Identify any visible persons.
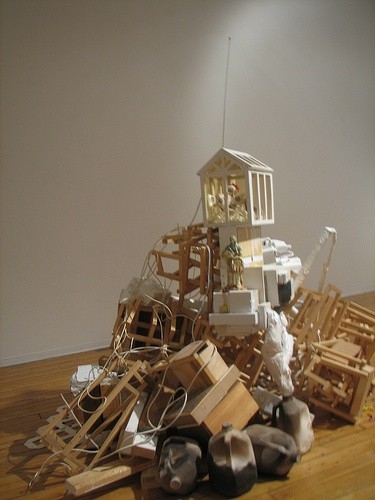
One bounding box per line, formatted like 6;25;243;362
222;235;245;291
